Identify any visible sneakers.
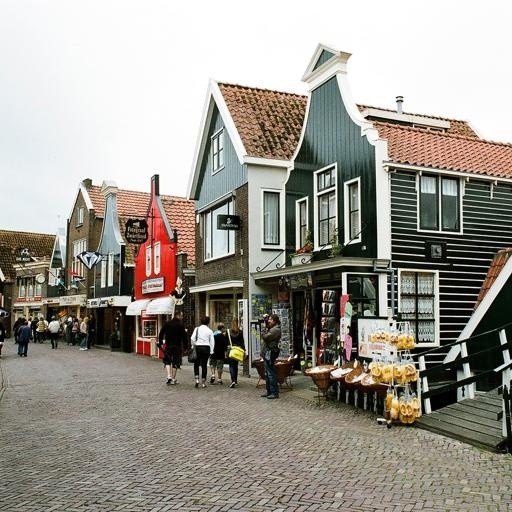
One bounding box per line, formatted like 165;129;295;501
195;377;238;388
165;377;177;384
79;347;88;351
260;394;279;399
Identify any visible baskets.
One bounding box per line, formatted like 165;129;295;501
305;362;376;393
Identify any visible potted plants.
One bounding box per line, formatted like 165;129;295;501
287;230;314;266
327;223;344;258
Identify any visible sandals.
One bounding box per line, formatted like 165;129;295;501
371;330;422;423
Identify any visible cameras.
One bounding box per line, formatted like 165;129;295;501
258;313;269;322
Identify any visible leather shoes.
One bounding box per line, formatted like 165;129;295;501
20;353;27;357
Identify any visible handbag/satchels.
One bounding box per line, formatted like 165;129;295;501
188;348;199;362
271;348;280;359
228;346;244;361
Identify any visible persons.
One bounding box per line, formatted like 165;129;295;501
159;310;189;385
261;314;281;399
0;312;96;359
169;277;186;319
191;317;245;388
